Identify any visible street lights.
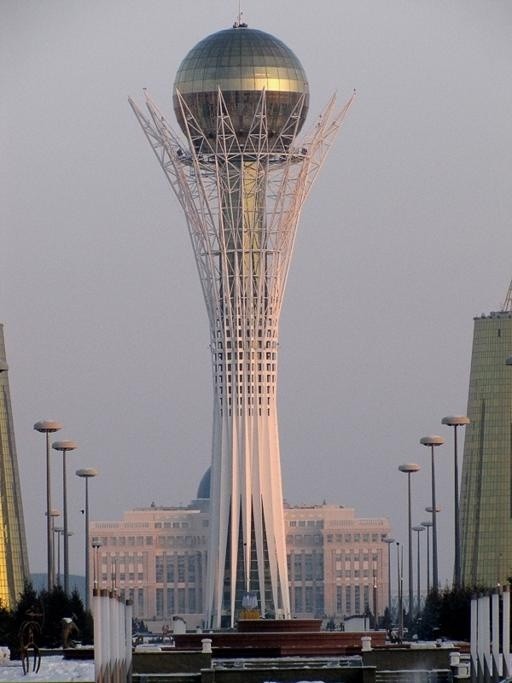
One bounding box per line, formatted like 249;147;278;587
33;420;98;612
381;415;469;633
